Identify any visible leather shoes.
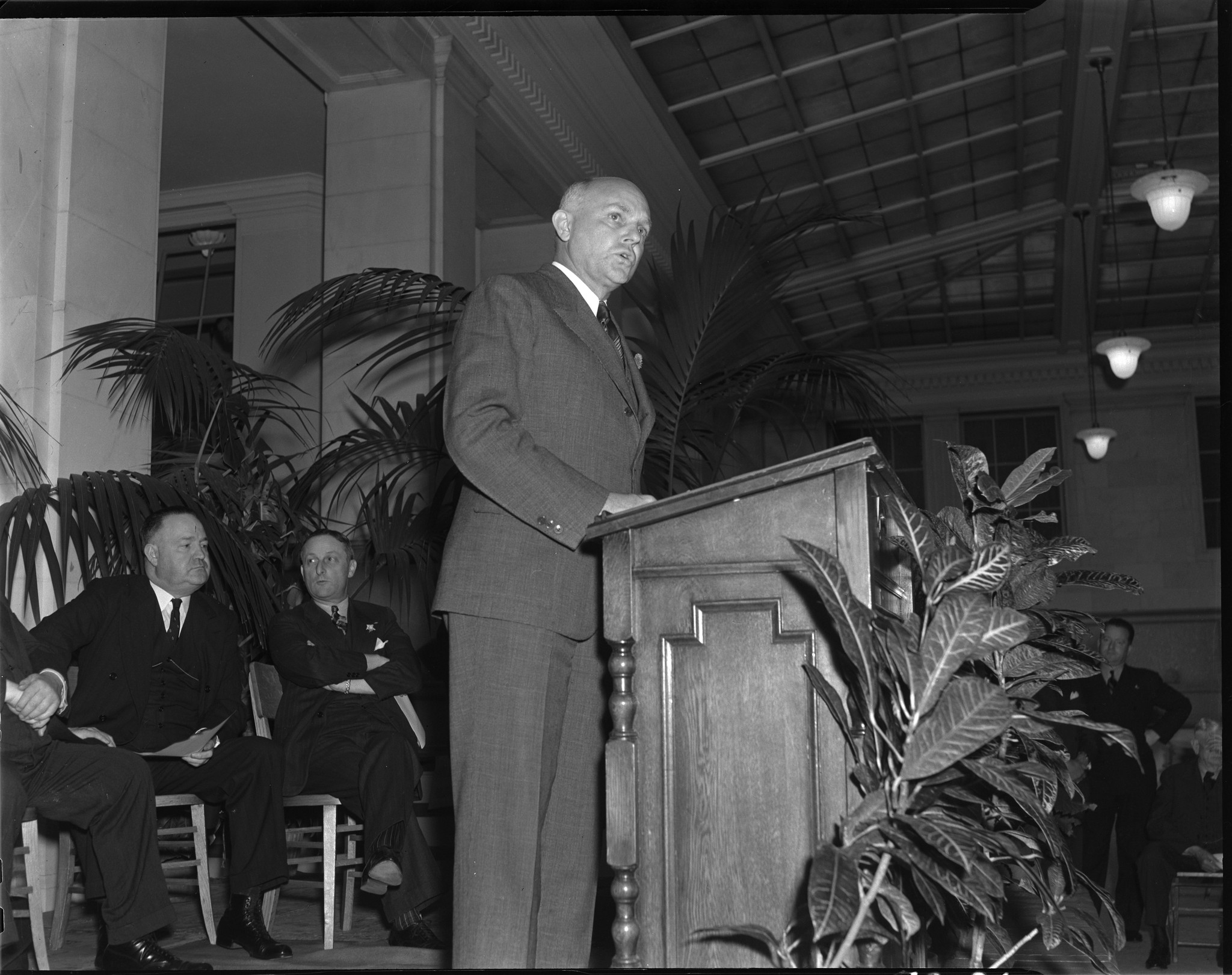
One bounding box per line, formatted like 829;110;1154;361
1145;943;1171;969
1125;930;1143;943
394;921;450;950
95;929;213;970
360;848;403;895
1216;946;1224;968
215;893;293;960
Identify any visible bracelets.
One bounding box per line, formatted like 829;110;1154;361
344;679;351;694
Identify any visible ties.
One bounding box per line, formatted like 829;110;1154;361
167;598;183;647
597;301;622;363
330;606;347;635
1204;771;1215;789
1106;670;1117;697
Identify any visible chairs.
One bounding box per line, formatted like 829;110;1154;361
246;657;366;953
9;804;50;970
45;664;218;950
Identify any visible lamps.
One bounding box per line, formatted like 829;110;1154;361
1127;0;1212;233
1088;54;1150;380
1067;202;1120;460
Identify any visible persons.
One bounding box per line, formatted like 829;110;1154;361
27;505;293;960
1141;718;1224;969
1062;618;1192;943
0;591;214;971
268;529;449;949
430;177;656;969
